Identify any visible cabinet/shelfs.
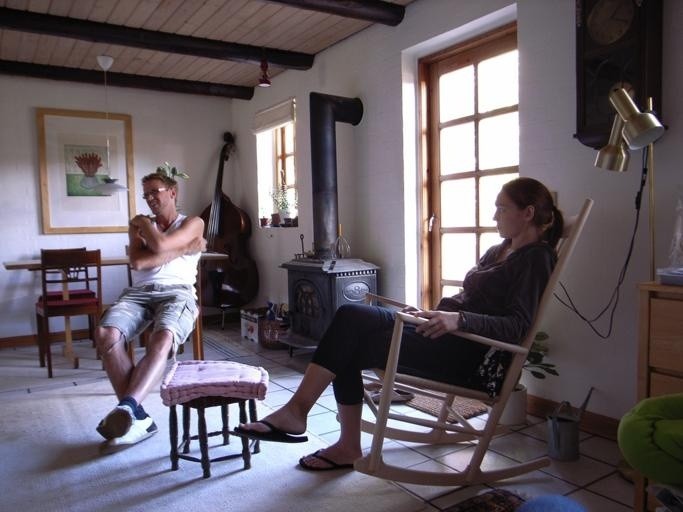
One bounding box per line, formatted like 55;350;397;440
634;278;682;512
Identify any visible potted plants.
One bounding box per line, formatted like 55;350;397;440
269;168;297;227
493;328;561;426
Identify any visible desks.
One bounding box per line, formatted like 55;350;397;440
2;249;230;378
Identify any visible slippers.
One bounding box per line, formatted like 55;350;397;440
234;420;308;443
299;449;353;471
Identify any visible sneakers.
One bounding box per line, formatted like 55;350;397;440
96;404;136;440
108;413;159;446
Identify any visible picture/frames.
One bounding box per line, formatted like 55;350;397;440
35;108;137;235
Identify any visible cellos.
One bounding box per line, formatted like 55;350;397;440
195;131;258;308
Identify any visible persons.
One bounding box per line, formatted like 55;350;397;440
92;172;208;448
234;174;565;471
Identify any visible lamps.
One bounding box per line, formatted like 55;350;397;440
258;59;271;87
594;85;664;483
87;56;130;196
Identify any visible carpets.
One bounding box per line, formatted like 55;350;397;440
440;489;525;512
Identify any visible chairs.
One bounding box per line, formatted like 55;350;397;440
32;246;102;378
40;247;92;342
335;191;592;490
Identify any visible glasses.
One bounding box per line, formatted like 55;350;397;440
143;187;169;199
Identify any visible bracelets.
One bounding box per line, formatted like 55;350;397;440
456;309;467;330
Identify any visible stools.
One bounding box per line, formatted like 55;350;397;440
168;395;260;478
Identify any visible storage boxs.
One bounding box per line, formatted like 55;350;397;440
239;303;288;349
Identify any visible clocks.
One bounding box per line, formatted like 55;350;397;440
572;2;665;152
